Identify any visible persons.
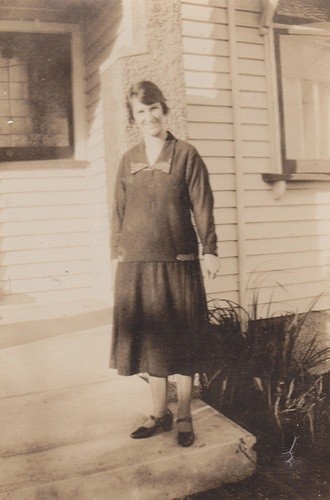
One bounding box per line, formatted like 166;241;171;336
109;81;222;448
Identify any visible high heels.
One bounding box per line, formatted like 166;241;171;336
130;409;173;439
176;418;194;447
